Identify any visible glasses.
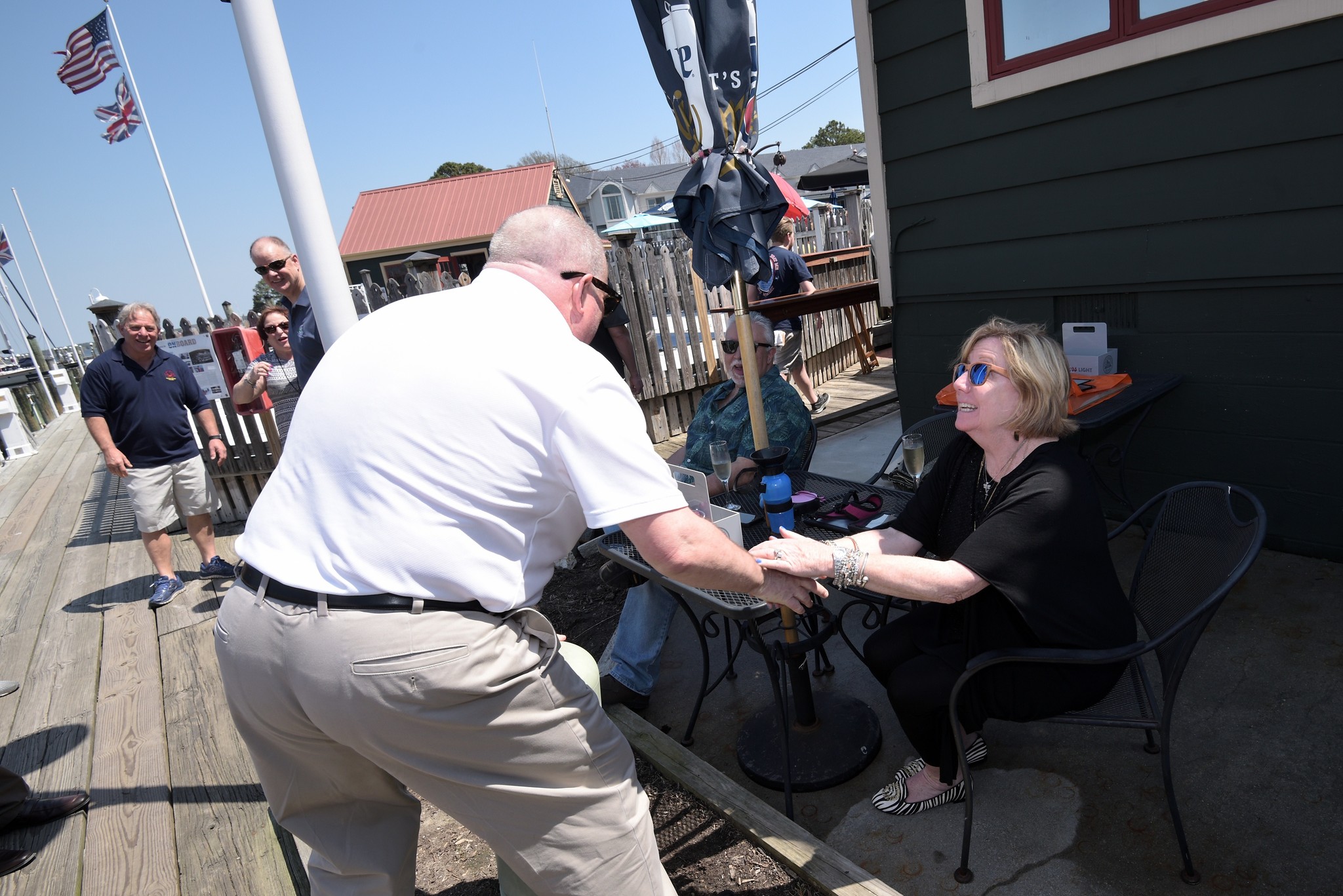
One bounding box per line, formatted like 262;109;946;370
263;321;290;334
953;362;1010;385
255;257;290;275
561;271;623;317
720;340;771;353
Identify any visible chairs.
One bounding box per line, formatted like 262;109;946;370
652;478;1268;888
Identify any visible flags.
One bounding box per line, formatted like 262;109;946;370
51;6;122;95
0;229;15;269
93;74;143;145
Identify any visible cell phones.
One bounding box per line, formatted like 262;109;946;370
732;509;764;527
847;510;900;534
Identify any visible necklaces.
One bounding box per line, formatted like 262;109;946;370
273;348;301;395
973;436;1031;534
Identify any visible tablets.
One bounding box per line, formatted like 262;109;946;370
803;516;856;536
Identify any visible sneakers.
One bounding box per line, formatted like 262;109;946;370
148;575;186;607
199;556;242;579
811;391;830;414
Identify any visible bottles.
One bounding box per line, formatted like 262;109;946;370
732;446;794;534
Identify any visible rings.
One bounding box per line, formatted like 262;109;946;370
774;547;781;560
257;368;259;372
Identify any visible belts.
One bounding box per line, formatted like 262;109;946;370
239;562;488;613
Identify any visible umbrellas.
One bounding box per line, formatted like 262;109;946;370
630;0;813;484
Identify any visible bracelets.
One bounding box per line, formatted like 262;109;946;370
821;536;870;590
245;372;256;386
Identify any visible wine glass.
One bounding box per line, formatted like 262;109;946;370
902;433;925;492
710;440;741;511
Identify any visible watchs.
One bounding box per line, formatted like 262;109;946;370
208;434;223;441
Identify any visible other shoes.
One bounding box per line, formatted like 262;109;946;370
599;559;649;588
871;772;974;815
895;736;988;781
599;674;648;712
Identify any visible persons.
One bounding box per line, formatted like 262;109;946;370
745;217;828;414
79;300;244;608
583;295;644;394
249;234;325;394
747;315;1137;818
231;304;302;463
598;310;817;710
211;205;829;895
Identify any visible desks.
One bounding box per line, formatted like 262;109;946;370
596;470;916;796
710;278;880;374
929;367;1187;545
